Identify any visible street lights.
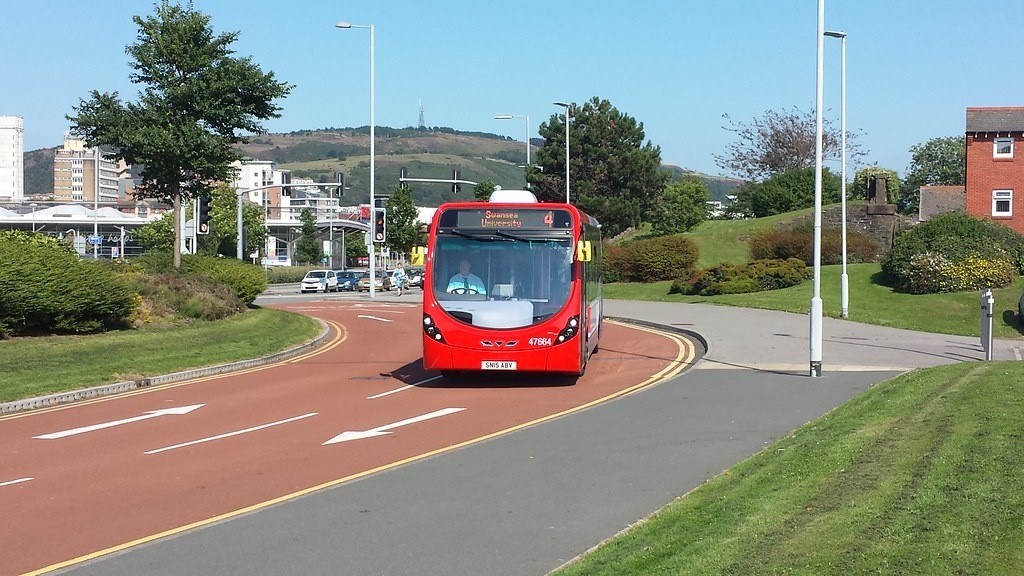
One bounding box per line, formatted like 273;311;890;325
493;115;531;193
825;30;849;320
332;228;344;269
29;203;37;232
336;22;377;299
553;102;571;203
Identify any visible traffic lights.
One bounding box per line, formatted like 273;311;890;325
196;195;212;235
373;208;386;243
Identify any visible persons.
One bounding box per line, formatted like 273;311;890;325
446;259;486;294
392;263;405;296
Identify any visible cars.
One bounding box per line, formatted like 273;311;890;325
357;270;392;293
335;271;365;291
387;267;424;290
300;270;338;294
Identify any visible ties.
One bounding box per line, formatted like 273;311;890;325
462;277;470;294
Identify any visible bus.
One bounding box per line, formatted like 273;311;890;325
412;190;604;379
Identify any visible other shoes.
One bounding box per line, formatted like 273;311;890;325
398;293;401;296
